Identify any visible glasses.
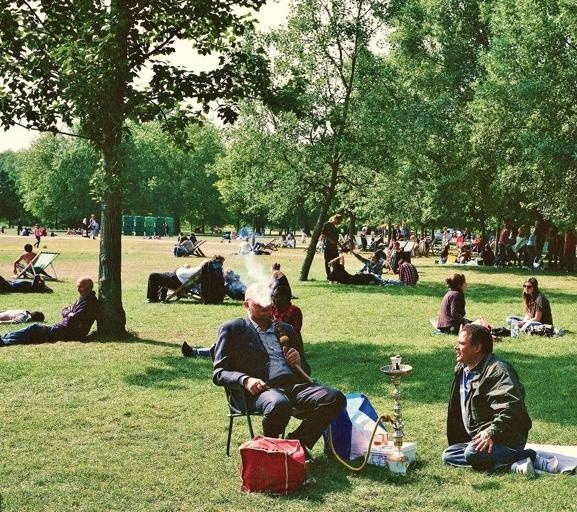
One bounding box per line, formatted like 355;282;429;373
523;285;533;288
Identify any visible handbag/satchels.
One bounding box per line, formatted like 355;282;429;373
239;434;305;496
323;393;389;471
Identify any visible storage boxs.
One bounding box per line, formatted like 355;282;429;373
364;441;416;466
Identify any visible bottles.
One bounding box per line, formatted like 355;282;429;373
511;320;519;337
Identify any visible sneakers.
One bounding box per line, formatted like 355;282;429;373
511;457;535;476
182;342;192;357
534;453;559;474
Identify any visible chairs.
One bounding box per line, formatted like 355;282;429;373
264;239;278;250
159;260;223;303
16;252;59;280
382;237;480;270
211;344;329;458
185;240;205;256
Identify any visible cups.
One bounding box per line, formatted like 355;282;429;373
380;433;389;447
390;357;403;370
373;435;383;446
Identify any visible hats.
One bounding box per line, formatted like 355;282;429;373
245;282;270;301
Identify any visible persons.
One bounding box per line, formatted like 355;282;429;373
442;227;451;251
67;228;76;235
360;227;382;251
147;254;225;302
83;215;99;239
162;223;169;236
327;255;384;285
268;262;289;286
456;230;496;266
0;307;44;324
302;230;307;244
318;214;348;276
223;225;296;254
17;221;30;236
353;242;418;285
495;224;542;270
14;244;48;276
441;322;559;475
436;272;514;336
33;224;47;248
391;222;430;257
504;277;554;334
211;285;345;460
549;229;574;270
173;233;197;257
182;284;302;356
0;276;96;347
